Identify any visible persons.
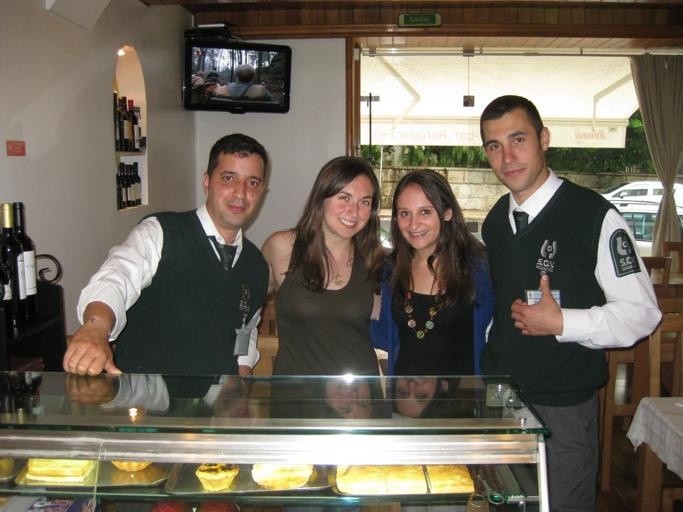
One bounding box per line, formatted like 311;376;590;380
63;133;270;376
214;65;272;100
260;156;386;377
192;71;204;102
64;377;269;434
392;376;462;418
369;168;496;374
482;95;663;512
309;378;386;418
196;66;220;101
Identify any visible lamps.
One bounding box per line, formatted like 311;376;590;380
462;51;475;108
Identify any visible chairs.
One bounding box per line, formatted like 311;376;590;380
639;317;683;512
599;296;683;441
663;242;681;275
638;256;671;297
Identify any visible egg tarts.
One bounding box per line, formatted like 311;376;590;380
111;466;164;484
197;460;239;492
114;460;151;472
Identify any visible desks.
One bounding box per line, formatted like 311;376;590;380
598;312;683;492
624;396;683;511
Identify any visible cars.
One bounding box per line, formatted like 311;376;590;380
562;200;683;258
600;182;683;215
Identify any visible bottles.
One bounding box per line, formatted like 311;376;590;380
0;260;12;328
116;163;141;210
111;93;141;153
0;371;42;424
0;204;28;329
8;204;37;323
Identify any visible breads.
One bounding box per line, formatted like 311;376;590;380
335;462;427;498
27;459;99;483
424;463;474;494
251;462;313;491
0;458;16;477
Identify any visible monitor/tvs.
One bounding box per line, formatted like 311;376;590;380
184;39;292;115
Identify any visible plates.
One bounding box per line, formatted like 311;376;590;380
329;464;481;500
14;464;170;488
164;463;337;497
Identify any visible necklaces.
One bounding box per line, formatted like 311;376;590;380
393;245;452;339
324;262;352;287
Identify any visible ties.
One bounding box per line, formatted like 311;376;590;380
207;235;238;272
512;210;529;234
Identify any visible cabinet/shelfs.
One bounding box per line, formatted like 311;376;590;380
1;372;549;512
